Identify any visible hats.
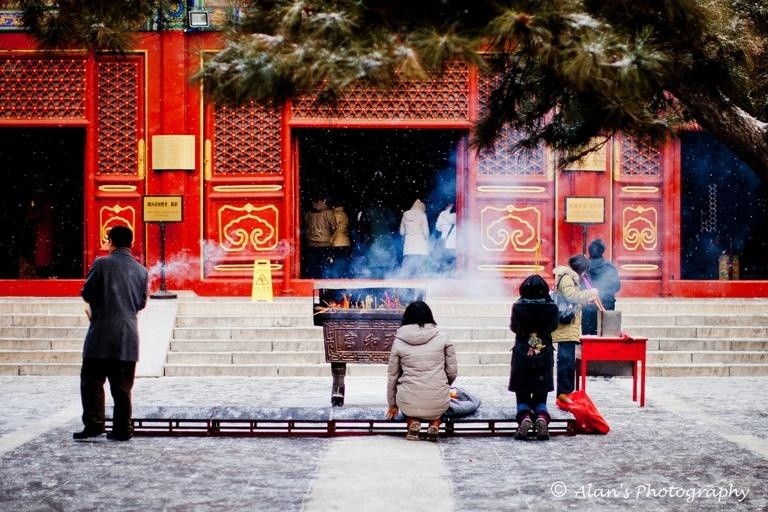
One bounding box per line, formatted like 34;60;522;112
413;200;426;212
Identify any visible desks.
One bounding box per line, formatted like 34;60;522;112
572;333;651;411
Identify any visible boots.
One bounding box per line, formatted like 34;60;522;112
534;410;550;440
426;420;441;442
514;409;534;439
407;417;421;440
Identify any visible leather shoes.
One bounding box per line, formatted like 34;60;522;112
73;428;132;441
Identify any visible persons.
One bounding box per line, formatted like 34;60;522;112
509;275;558;439
550;254;596;397
386;301;457;441
73;226;149;441
581;239;621;380
1;202;84;280
301;193;456;279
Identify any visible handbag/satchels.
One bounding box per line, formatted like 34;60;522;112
430;238;445;261
450;386;481;414
556;390;610;434
550;287;577;325
